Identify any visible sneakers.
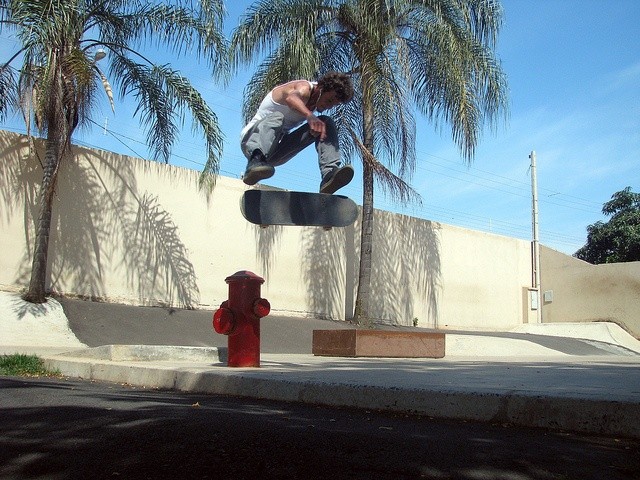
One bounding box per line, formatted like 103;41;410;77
243;156;274;184
320;165;354;194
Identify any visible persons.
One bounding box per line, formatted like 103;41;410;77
239;72;354;194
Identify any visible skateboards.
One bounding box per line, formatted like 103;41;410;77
239;189;358;231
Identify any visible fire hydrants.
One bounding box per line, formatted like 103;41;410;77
213;271;270;367
